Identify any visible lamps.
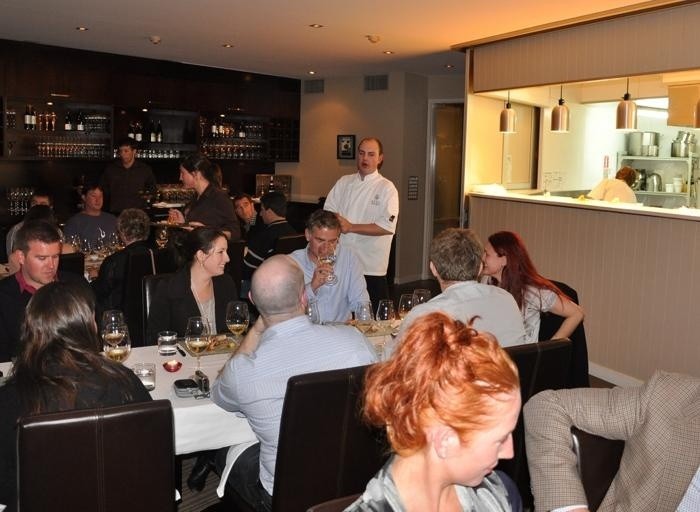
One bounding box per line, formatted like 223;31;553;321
551;84;570;132
615;76;637;129
499;89;519;134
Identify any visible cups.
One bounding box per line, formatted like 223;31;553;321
666;178;683;193
157;329;178;358
399;289;430;322
134;364;157;392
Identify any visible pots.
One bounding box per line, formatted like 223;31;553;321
628;132;659;157
671;143;696;157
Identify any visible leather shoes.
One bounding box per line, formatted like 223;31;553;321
187;455;215;491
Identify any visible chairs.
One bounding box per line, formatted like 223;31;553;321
112;233;306;347
571;425;625;512
14;400;176;512
221;364;391;512
57;252;84;280
502;280;590;458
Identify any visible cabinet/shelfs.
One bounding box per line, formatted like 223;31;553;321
617;154;692;207
0;96;114;163
201;112;270;160
115;105;201;158
271;117;300;161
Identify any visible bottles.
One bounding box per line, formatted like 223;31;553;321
269;176;275;192
282;184;289;201
7;103;264;161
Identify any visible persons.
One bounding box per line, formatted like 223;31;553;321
168;152;241;241
203;255;383;511
588;167;639;202
323;137;399;300
483;231;584;340
7;186;74;258
395;228;522;356
0;281;155;511
90;208;169;346
64;183;118;249
148;225;238;344
523;371;700;512
345;311;523;512
240;192;299;279
0;220;96;362
99;137;158;216
293;210;374;326
234;195;265;236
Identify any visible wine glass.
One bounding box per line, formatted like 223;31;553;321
305;303;320;324
376;299;396;346
184;317;211;380
102;310;130;365
34;141;264;161
317;242;338;286
8;190;34;215
354;302;373;347
225;302;250;361
71;229;122;259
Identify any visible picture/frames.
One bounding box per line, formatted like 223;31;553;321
337;135;355;159
